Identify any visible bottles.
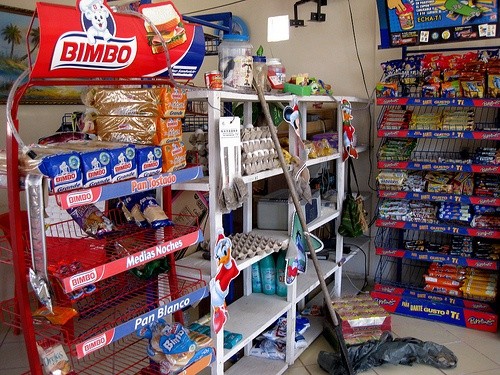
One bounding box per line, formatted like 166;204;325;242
266;57;287;95
251;55;268;95
259;254;276;295
217;34;254;94
251;262;263;293
276;248;287;297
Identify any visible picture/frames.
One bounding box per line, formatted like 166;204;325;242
0;4;90;106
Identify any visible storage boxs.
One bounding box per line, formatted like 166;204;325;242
323;294;392;355
256;188;322;232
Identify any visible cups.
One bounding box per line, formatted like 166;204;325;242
204;70;224;91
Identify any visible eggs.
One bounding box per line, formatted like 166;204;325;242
187;125;287;261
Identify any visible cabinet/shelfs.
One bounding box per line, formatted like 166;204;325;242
169;94;373;374
371;47;500;334
0;79;217;375
